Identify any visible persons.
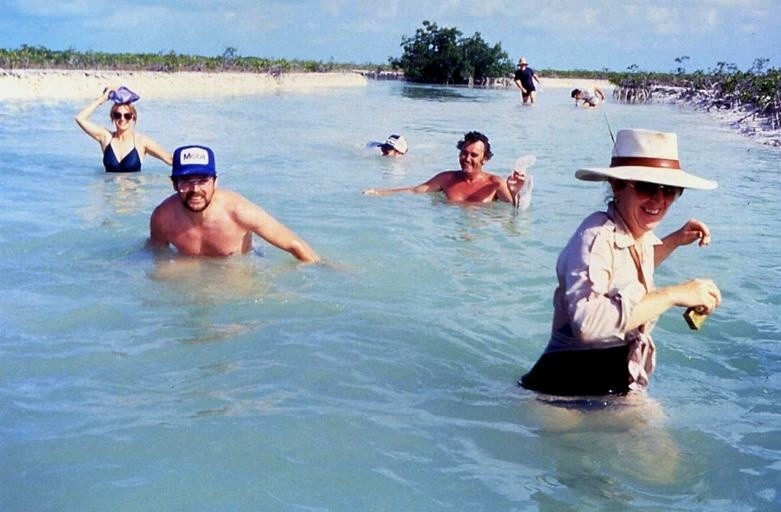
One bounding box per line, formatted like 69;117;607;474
516;126;720;487
363;133;531;215
143;146;325;264
375;136;410;157
75;90;178;172
571;86;609;108
516;58;542;105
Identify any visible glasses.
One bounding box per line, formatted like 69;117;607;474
113;111;133;120
624;181;685;202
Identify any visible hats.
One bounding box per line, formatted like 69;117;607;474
171;146;216;178
517;58;528;66
377;134;406;155
575;128;719;191
